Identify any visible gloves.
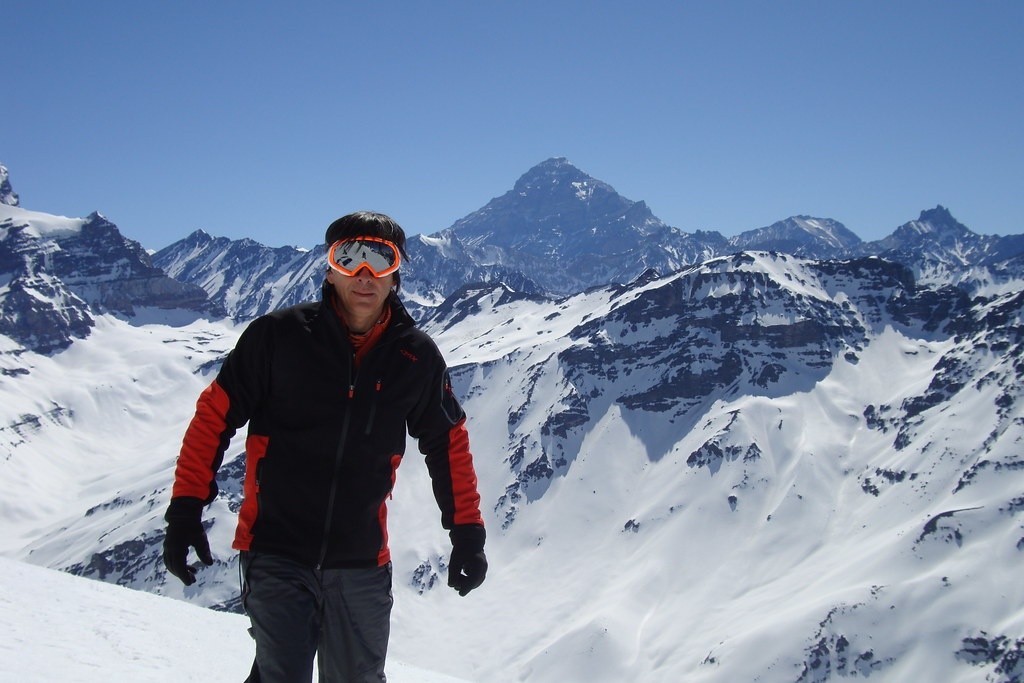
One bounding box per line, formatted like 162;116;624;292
162;502;213;586
448;525;488;596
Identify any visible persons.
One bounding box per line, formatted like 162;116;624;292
163;211;487;683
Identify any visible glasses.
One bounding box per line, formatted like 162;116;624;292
328;235;401;278
